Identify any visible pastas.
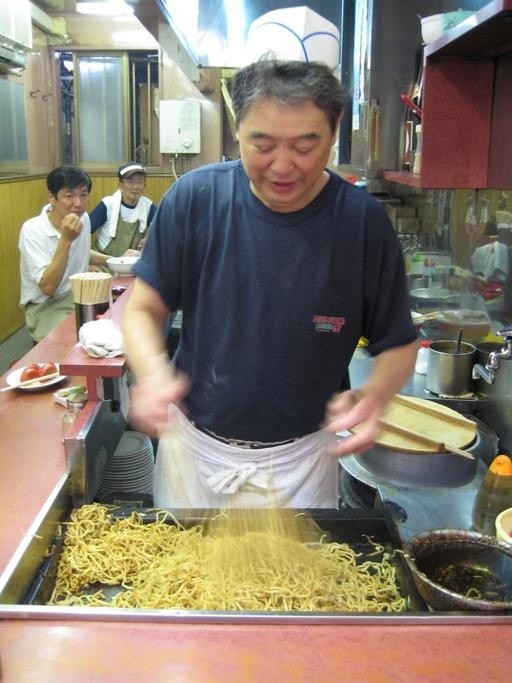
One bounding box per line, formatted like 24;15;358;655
46;502;408;613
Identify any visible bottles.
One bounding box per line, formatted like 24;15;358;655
416;338;429;376
410;256;424;273
471;453;512;534
402;46;420;171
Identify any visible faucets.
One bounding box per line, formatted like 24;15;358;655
472;330;512;383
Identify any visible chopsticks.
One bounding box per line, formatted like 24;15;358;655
0;372;62;392
69;272;113;305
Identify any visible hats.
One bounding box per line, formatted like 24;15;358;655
246;7;342;71
118;163;146;179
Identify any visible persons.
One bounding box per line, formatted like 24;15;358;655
88;161;158;274
122;57;422;507
19;167;101;345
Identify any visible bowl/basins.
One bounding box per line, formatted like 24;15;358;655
404;527;512;610
420;9;470;45
106;256;141;276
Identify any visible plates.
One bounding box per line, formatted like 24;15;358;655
6;364;70;394
96;430;154;495
